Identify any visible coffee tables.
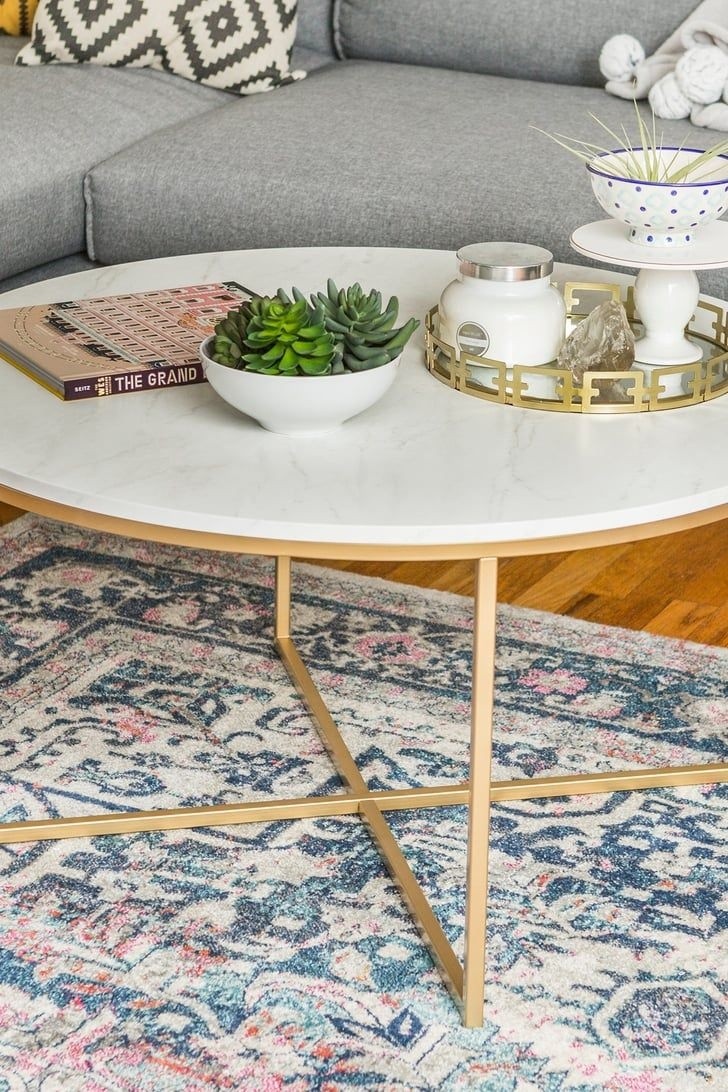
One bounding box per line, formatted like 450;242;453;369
0;247;728;1027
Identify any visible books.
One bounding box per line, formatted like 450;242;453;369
0;281;264;401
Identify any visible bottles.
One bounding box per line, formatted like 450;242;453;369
438;242;564;370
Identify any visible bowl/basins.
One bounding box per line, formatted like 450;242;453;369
585;145;728;245
200;335;405;436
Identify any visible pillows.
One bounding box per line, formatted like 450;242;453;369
0;0;307;94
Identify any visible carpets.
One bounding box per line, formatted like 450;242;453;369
0;510;728;1092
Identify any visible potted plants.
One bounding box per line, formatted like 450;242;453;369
199;277;420;433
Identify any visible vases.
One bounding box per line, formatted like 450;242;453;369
585;148;728;247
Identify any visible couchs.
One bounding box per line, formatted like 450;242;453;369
0;0;728;302
